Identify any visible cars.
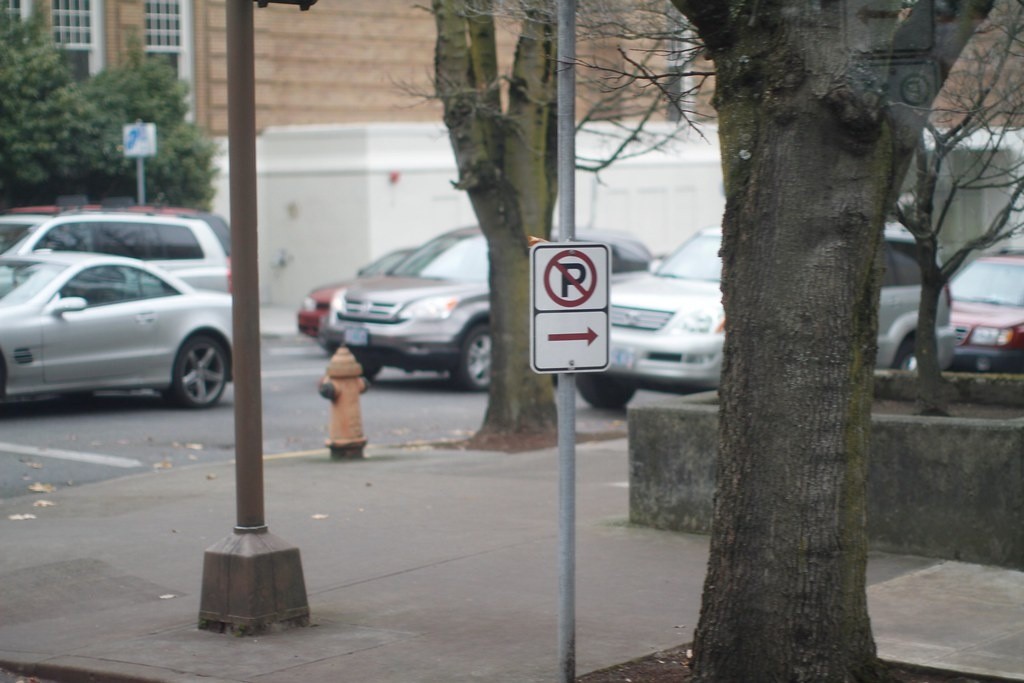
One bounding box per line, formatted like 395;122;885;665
948;256;1023;372
1;251;232;408
316;227;650;392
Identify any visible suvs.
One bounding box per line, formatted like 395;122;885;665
1;213;232;293
573;224;955;407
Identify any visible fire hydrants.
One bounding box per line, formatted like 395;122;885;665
319;342;371;456
